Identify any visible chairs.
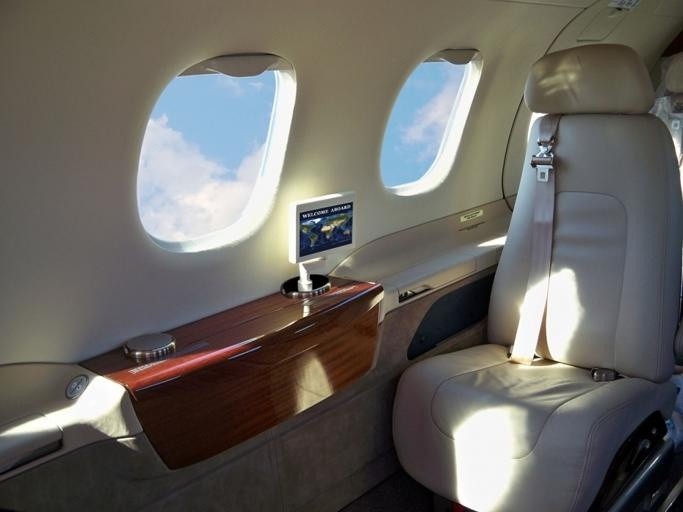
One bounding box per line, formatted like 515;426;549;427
648;52;683;198
393;43;683;512
